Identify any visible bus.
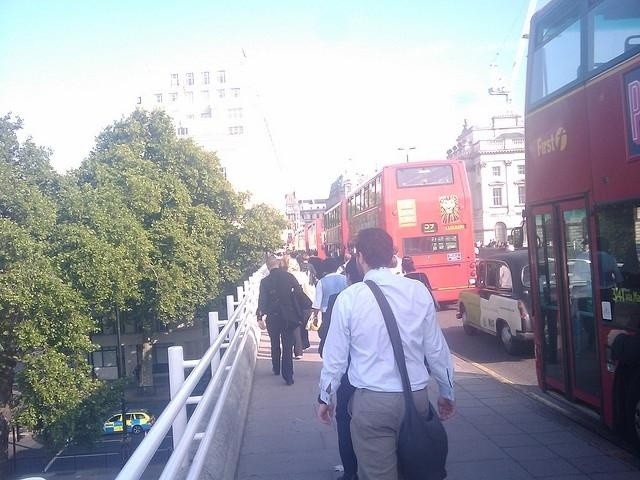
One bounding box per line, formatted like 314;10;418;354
288;160;478;306
525;0;640;449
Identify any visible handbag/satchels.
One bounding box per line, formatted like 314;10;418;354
395;400;448;480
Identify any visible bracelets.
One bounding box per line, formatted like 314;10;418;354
314;316;317;320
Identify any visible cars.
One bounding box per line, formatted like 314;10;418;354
103;408;156;435
455;250;536;355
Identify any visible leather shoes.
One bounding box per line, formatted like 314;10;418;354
337;473;358;480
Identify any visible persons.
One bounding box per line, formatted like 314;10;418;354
605;328;639;362
474;239;508;255
287;257;310;360
278;246;353;288
574;235;624;342
313;259;348;328
620;247;640;289
255;254;303;385
313;223;457;480
402;256;441;312
318;257;365;480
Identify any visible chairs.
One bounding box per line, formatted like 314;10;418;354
577;33;640;79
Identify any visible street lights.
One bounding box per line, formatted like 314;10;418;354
398;146;416;162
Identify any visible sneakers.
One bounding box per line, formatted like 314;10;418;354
272;352;304;386
